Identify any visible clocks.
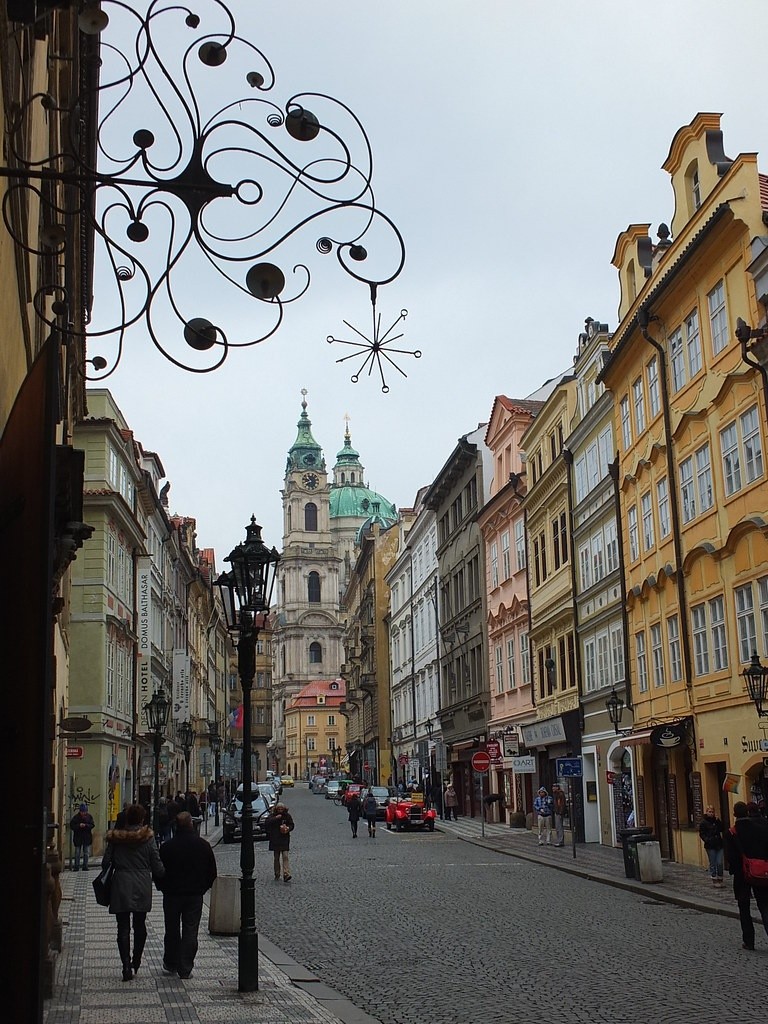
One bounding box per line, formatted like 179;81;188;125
302;472;320;489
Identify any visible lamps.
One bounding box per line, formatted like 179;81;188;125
738;651;768;718
604;684;626;735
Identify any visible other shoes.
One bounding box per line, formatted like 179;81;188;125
72;868;79;872
554;843;563;846
82;868;90;871
163;963;178;973
179;974;193;979
741;942;754;950
284;875;292;882
275;874;280;880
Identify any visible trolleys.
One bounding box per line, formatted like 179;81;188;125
334;789;346;806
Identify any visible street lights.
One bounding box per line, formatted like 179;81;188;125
209;511;282;994
336;744;342;771
330;746;337;762
211;734;225;826
176;717;198;794
140;683;172;881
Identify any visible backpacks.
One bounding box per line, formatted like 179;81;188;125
367;797;376;811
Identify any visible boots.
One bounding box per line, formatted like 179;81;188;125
711;876;721;887
717;878;726;887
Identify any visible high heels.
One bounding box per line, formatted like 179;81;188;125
130;958;141;974
122;966;132;982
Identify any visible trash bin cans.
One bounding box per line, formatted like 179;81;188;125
620;827;664;884
207;873;241;936
192;817;202;837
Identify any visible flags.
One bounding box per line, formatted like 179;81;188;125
228;705;243;728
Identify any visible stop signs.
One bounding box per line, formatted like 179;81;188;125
471;751;492;773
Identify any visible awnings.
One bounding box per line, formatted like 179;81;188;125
619;722;682;748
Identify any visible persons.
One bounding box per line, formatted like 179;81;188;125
482;792;506;823
70;804;95;872
532;786;555;846
726;801;768;951
698;804;726;887
265;802;295;882
552;783;567;846
388;774;459;821
152;812;218;978
347;794;362;838
363;794;378;838
145;774;238;845
101;804;166;982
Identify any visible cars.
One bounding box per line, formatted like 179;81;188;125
308;773;345;799
344;784;399;819
220;781;279;843
266;768;295;795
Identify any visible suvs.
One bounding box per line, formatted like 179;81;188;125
384;790;437;832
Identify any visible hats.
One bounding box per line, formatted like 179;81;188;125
125;804;146;818
79;804;89;813
351;794;358;798
746;802;758;811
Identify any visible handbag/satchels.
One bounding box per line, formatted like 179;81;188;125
92;860;114;907
743;858;768;884
